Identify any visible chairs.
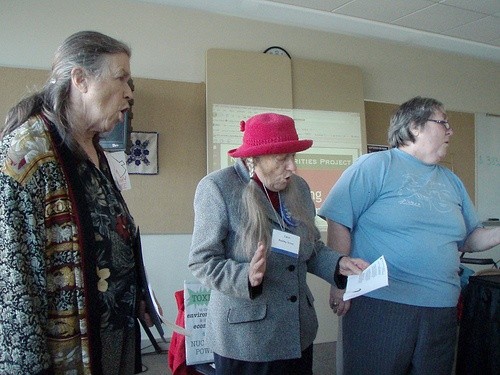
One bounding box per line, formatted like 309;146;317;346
174;289;216;375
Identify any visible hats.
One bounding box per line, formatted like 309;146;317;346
228;113;313;157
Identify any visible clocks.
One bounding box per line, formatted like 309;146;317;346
263;46;291;59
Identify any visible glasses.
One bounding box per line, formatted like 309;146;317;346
429;119;449;130
